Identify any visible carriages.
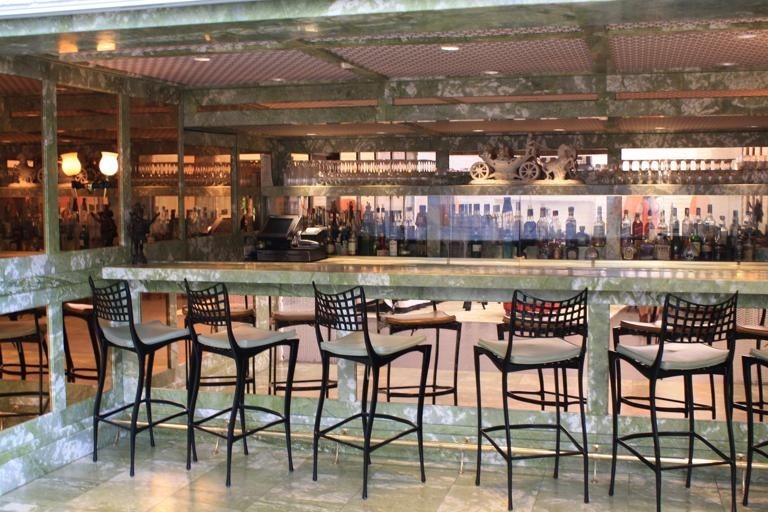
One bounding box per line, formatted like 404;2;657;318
13;152;91;185
471;136;577;180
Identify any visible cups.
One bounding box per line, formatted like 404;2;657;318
638;305;659;323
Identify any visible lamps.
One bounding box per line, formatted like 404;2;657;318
59;150;82;177
99;150;119;177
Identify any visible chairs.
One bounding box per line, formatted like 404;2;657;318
88;275;203;476
312;281;433;501
472;287;590;509
609;290;765;512
0;301;101;429
184;294;588;414
182;278;299;487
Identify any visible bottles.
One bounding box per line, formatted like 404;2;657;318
299;200;426;257
442;197;606;263
621;197;768;262
1;196;263;256
742;147;768;169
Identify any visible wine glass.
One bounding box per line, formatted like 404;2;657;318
128;160;261;187
579;158;737;185
279;156;469;187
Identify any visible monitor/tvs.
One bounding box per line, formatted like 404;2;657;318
258;214;302;241
